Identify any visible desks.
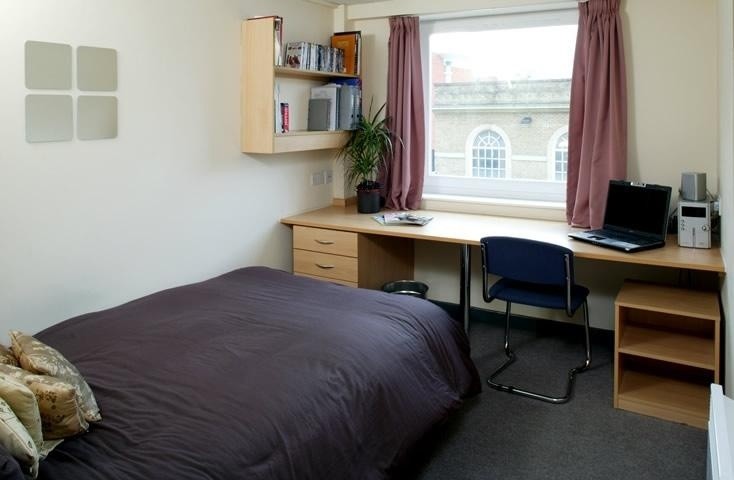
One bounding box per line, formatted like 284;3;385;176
280;203;726;288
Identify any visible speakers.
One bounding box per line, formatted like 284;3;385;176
683;174;707;202
678;202;711;248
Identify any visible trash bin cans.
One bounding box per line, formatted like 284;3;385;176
382;278;431;297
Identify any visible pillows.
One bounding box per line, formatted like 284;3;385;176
0;395;40;479
9;329;103;423
0;343;19;367
0;376;44;447
1;362;90;441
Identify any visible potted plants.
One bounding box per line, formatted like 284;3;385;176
334;93;408;215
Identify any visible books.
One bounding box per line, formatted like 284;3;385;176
373;212;433;227
245;16;361;76
273;79;362;133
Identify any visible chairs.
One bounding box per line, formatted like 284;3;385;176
478;235;595;403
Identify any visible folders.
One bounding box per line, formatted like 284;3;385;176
247;14;284;67
340;85;360;131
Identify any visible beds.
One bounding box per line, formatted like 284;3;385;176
1;265;483;479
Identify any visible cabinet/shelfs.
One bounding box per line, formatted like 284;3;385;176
241;17;364;155
612;279;722;430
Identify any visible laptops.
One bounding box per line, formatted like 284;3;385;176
569;180;671;254
